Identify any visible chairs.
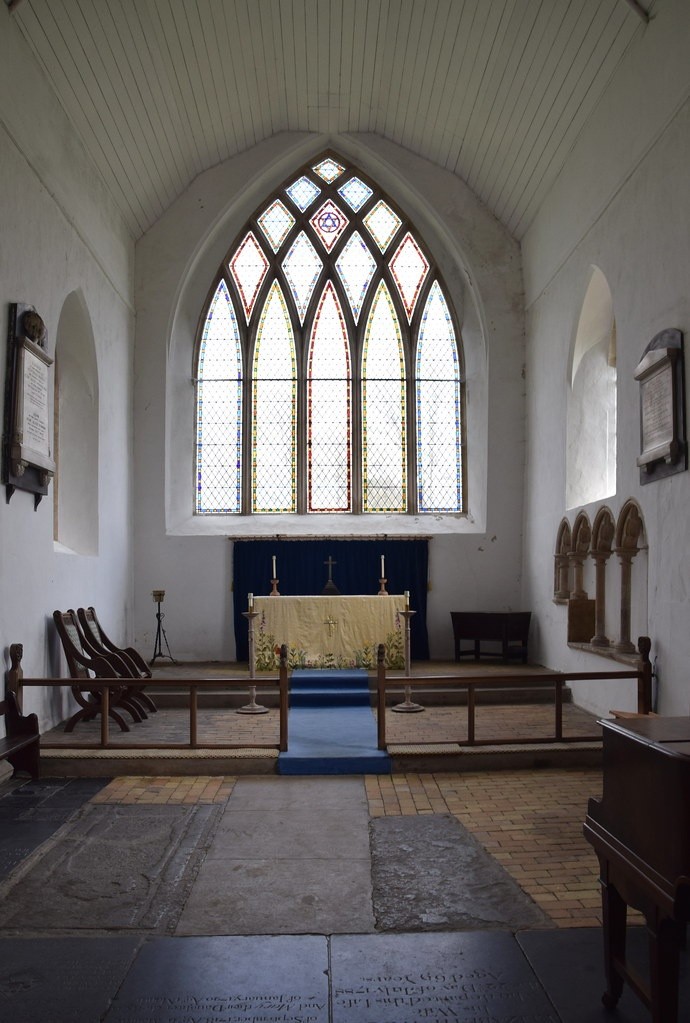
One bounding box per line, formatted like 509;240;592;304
53;603;162;735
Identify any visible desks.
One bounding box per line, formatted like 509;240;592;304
447;605;537;668
254;595;406;671
582;716;690;1023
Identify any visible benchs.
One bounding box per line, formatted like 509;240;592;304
0;690;40;782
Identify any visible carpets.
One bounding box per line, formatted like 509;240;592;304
279;665;391;775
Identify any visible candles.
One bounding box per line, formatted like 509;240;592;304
404;591;410;605
272;556;276;578
248;593;253;606
381;555;384;578
